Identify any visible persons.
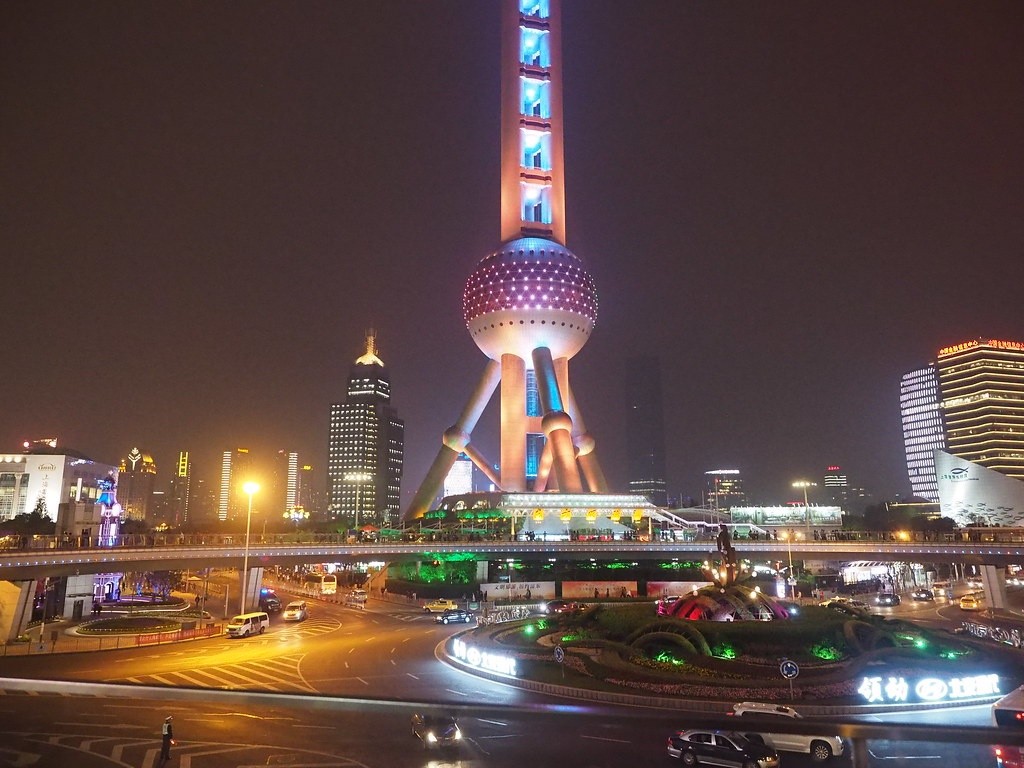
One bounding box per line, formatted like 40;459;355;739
0;518;1024;629
160;716;173;760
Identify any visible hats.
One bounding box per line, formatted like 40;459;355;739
165;716;173;721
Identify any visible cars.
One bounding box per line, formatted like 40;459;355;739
410;711;462;750
475;598;588;625
959;569;1024;611
912;588;934;601
655;596;682;605
432;609;474;625
819;595;870;615
876;593;900;607
666;729;781;768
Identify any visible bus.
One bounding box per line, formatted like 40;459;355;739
304;572;337;596
991;684;1024;767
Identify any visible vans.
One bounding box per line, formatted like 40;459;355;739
227;611;270;638
259;588;283;614
282;601;307;622
932;582;953;597
345;590;368;603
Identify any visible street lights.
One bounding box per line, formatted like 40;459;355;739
792;481;818;531
345;473;372;529
238;480;260;617
781;530;804;602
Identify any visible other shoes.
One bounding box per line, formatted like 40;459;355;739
161;757;172;760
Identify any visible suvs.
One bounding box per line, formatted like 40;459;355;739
422;597;457;615
727;701;845;764
